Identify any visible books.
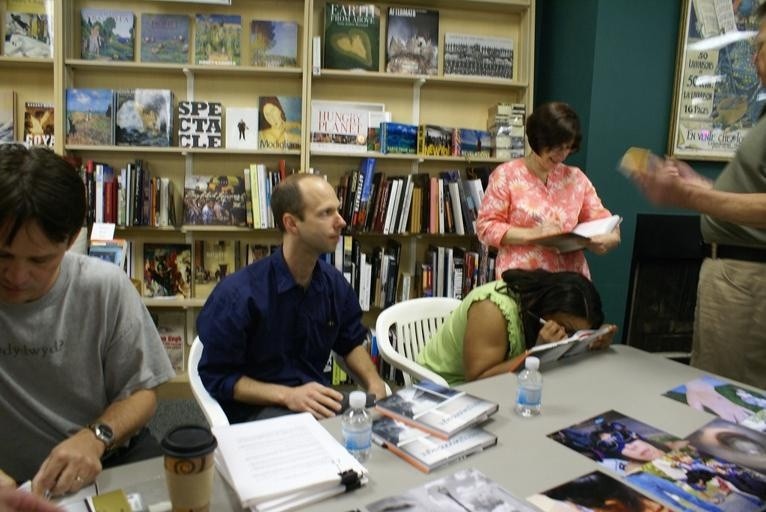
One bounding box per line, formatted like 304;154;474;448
67;159;496;391
372;379;500;442
0;3;528;161
614;144;714;190
368;414;498;474
531;213;625;259
61;487;132;512
209;408;366;512
215;464;371;512
508;324;617;370
363;465;541;511
211;450;347;511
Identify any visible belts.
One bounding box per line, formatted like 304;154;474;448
703;243;766;263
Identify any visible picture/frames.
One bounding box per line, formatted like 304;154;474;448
666;0;766;163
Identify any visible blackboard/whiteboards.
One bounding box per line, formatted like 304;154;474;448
621;213;700;353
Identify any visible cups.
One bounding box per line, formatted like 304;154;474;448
160;425;218;511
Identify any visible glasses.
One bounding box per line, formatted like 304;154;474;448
552;143;578;154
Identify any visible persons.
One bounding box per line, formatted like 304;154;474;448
473;100;621;281
637;0;765;389
1;139;177;512
647;449;765;503
541;470;672;512
194;169;394;426
563;417;689;474
406;264;603;391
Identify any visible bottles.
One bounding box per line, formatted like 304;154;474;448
516;356;546;416
342;389;374;469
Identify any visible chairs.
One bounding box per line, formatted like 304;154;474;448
375;296;462;390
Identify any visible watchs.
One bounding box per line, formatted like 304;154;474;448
87;417;116;457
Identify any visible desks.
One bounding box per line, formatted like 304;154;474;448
2;344;765;512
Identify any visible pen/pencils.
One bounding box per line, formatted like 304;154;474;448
527;309;546;325
